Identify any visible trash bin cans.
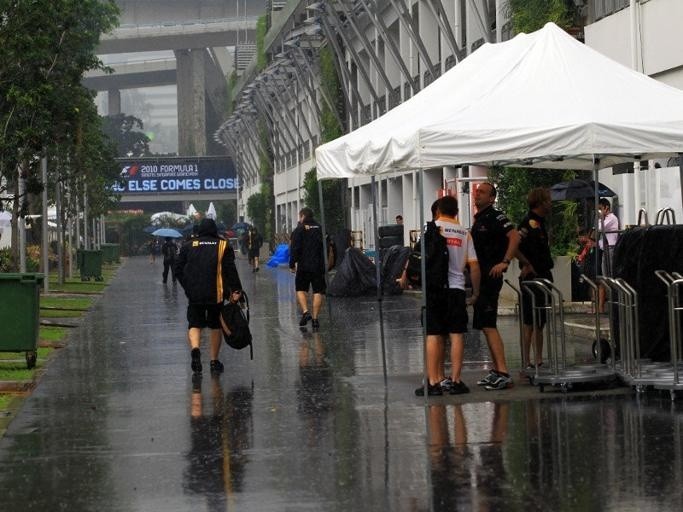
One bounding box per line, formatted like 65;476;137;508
76;248;104;281
102;244;114;264
0;272;44;369
112;244;120;264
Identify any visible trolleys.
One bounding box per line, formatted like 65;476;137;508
505;269;683;401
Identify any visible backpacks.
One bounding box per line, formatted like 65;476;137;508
426;222;450;291
407;242;423;287
219;288;253;361
227;387;253;450
166;246;176;261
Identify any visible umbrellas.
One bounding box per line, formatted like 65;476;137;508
547;176;615;199
183;224;196;231
150;229;183;237
142;226;157;234
230;223;249;229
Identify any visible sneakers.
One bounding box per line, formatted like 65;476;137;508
449;380;470;396
190;347;203;373
484;375;514;391
415;382;443;396
311;319;321;328
520;364;535;373
477;368;496;385
210;360;223;371
299;311;312;327
439;378;452;390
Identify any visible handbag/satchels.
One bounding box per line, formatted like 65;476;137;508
178;266;210;304
405;222;450;290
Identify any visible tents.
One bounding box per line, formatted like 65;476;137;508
313;20;683;386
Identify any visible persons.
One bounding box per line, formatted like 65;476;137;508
396;198;454;391
289;208;329;329
526;401;559;512
475;402;510;511
172;219;241;374
150;238;156;264
293;333;336;447
591;198;619;314
162;238;178;284
396;215;403;225
469;183;522;392
427;405;472;512
186;225;199;242
516;187;554;373
185;376;248;511
248;228;263;274
415;196;480;396
